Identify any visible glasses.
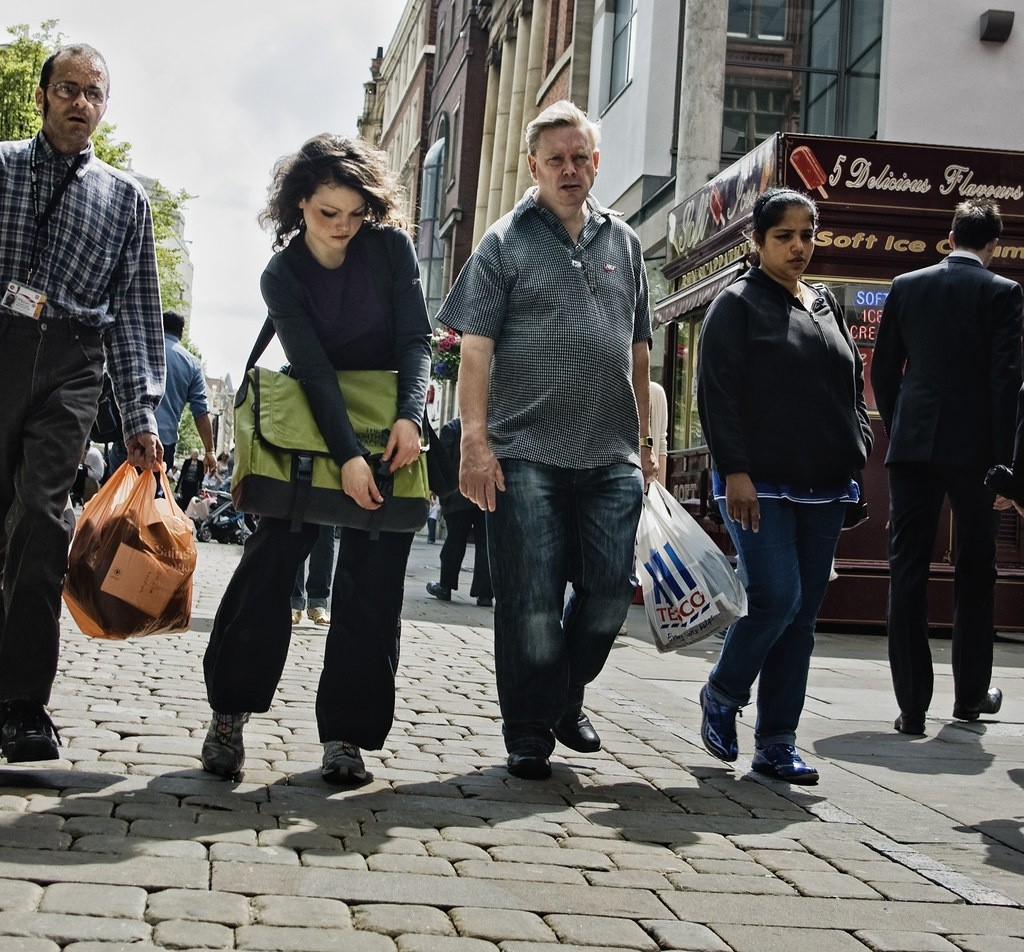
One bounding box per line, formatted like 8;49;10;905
47;82;108;104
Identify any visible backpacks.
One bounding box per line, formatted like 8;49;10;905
91;373;124;443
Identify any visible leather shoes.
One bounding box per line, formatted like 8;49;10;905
895;711;926;734
508;745;552;781
952;687;1003;719
554;709;600;753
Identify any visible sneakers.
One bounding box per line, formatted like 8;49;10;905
700;683;742;761
322;740;366;783
292;608;302;624
0;699;62;762
752;744;819;781
307;607;330;623
202;712;250;774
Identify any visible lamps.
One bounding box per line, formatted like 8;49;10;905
979;9;1014;41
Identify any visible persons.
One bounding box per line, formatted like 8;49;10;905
435;97;660;779
202;132;431;783
0;45;164;764
870;196;1023;734
69;312;666;607
696;188;874;784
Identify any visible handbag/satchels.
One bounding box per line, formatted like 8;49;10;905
636;479;748;653
231;366;430;532
842;469;869;531
66;459;195;639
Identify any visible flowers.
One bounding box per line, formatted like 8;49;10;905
429;327;460;383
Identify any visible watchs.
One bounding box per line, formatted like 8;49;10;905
639;437;654;448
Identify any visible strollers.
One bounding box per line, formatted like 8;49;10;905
195;475;251;545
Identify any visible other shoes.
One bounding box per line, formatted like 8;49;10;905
427;582;451;600
476;596;493;606
